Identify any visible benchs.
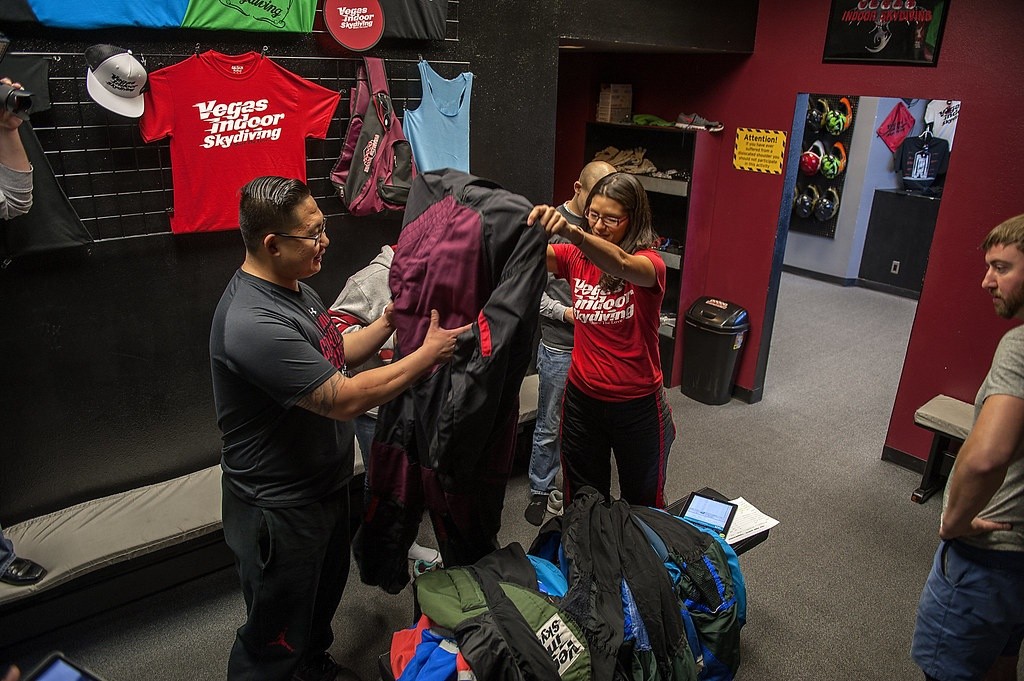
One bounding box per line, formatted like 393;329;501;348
911;394;977;504
378;486;768;681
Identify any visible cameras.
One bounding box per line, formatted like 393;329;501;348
0;82;36;121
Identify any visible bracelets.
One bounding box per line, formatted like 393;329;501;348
577;226;585;247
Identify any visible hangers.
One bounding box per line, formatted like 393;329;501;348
918;126;935;138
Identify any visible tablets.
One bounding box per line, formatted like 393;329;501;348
678;491;737;539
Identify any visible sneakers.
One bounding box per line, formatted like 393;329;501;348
676;111;725;133
548;489;565;516
411;559;445;578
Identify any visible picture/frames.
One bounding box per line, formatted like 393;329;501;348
821;0;950;66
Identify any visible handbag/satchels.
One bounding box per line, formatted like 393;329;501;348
335;56;419;219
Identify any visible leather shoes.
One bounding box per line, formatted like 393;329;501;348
0;555;49;588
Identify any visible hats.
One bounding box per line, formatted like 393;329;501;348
81;44;149;118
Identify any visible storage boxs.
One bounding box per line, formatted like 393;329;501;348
597;83;632;123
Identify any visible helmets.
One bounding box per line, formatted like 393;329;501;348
807;97;832;134
795;139;827;178
823;97;855;136
817;141;848;180
795;183;819;220
814;186;840;221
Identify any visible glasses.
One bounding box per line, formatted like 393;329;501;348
268;216;331;244
583;206;630;228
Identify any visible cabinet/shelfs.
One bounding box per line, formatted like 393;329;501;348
586;119;716;388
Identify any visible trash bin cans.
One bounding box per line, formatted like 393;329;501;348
685;295;750;405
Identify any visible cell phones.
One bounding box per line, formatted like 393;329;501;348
22;653;107;681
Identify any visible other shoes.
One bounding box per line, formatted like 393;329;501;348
298;652;367;681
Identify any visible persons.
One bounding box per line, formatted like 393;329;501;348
523;161;616;524
910;214;1024;681
328;244;444;561
209;177;477;680
0;77;47;584
527;171;676;510
